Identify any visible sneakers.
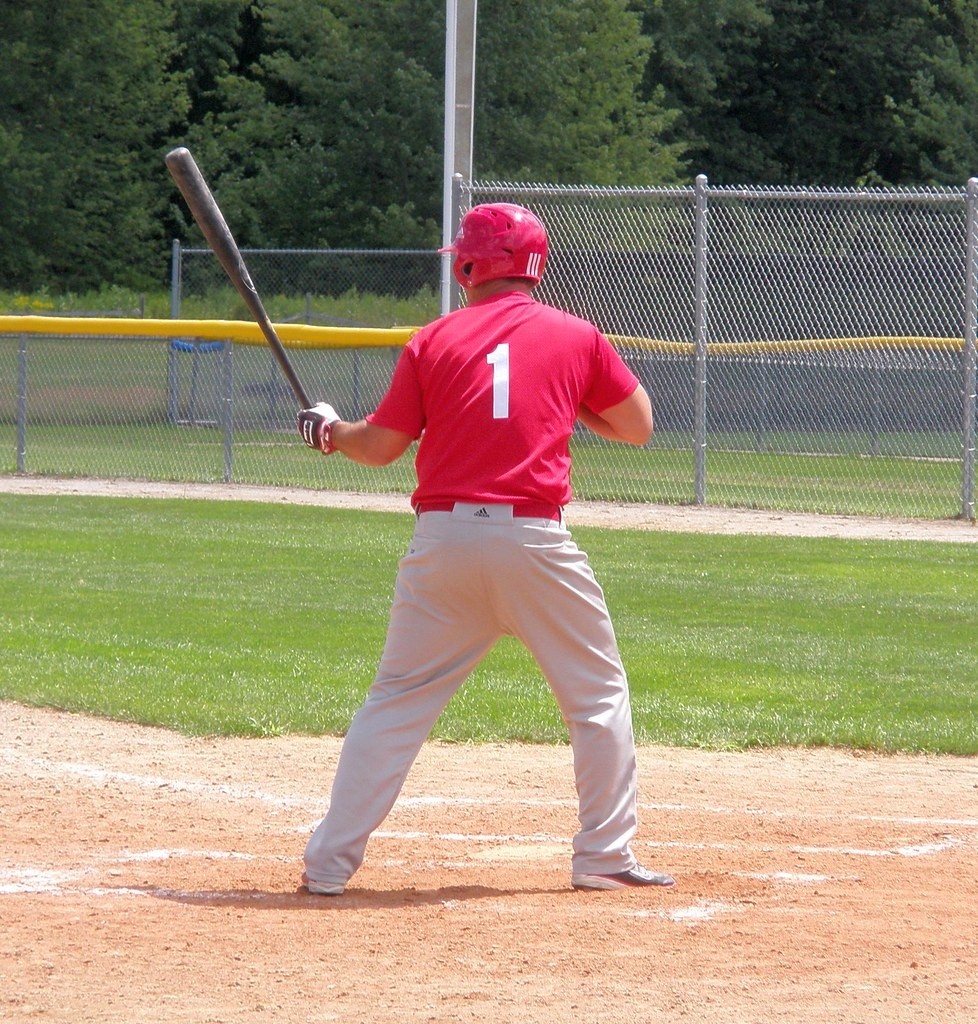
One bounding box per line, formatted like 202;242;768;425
571;863;675;890
302;872;345;895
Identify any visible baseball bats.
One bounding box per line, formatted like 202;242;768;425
164;146;314;413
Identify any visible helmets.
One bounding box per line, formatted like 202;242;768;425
435;203;548;289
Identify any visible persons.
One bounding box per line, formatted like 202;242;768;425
297;203;676;894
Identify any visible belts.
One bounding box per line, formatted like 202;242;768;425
417;502;561;522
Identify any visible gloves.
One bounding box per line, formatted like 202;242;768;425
296;402;341;455
